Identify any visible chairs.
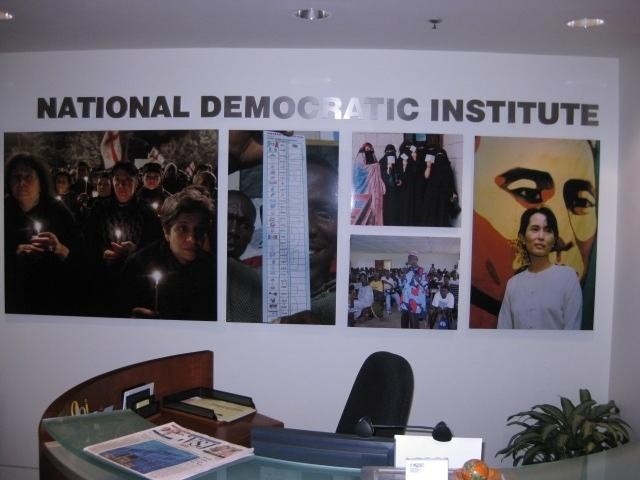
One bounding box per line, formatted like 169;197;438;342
334;347;415;441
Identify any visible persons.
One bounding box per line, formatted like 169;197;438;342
348;248;459;330
3;131;218;321
351;139;460;228
228;132;338;324
470;136;599;329
496;208;584;330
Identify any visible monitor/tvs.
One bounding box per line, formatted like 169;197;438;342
250;426;397;469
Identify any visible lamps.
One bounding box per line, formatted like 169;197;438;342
355;415;455;442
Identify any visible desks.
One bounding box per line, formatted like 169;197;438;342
36;350;639;480
